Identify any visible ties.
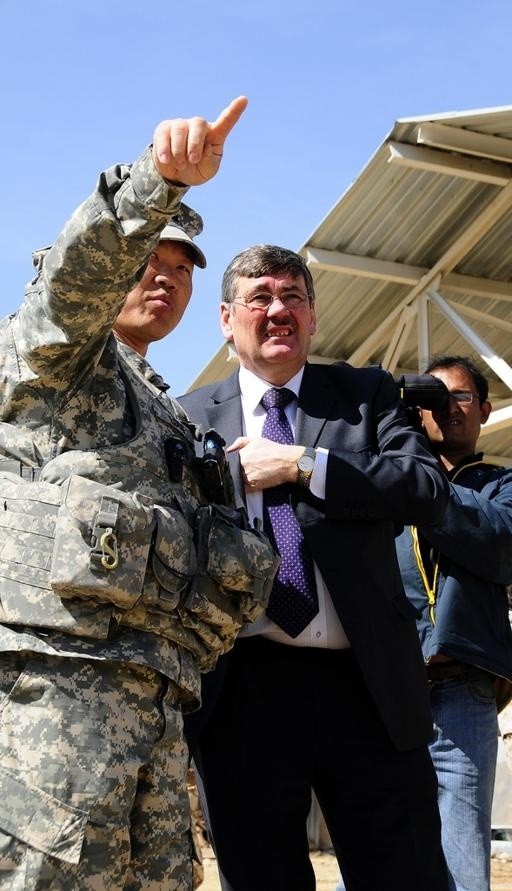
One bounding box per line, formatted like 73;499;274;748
259;388;319;640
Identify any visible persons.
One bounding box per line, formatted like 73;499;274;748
159;244;462;889
1;92;285;889
387;352;511;891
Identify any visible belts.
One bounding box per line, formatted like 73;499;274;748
424;663;484;682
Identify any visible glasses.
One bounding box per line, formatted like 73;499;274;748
227;290;314;308
449;391;483;405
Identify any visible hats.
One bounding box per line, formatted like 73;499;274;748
158;201;207;270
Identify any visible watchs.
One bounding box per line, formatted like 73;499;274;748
295;446;316;487
